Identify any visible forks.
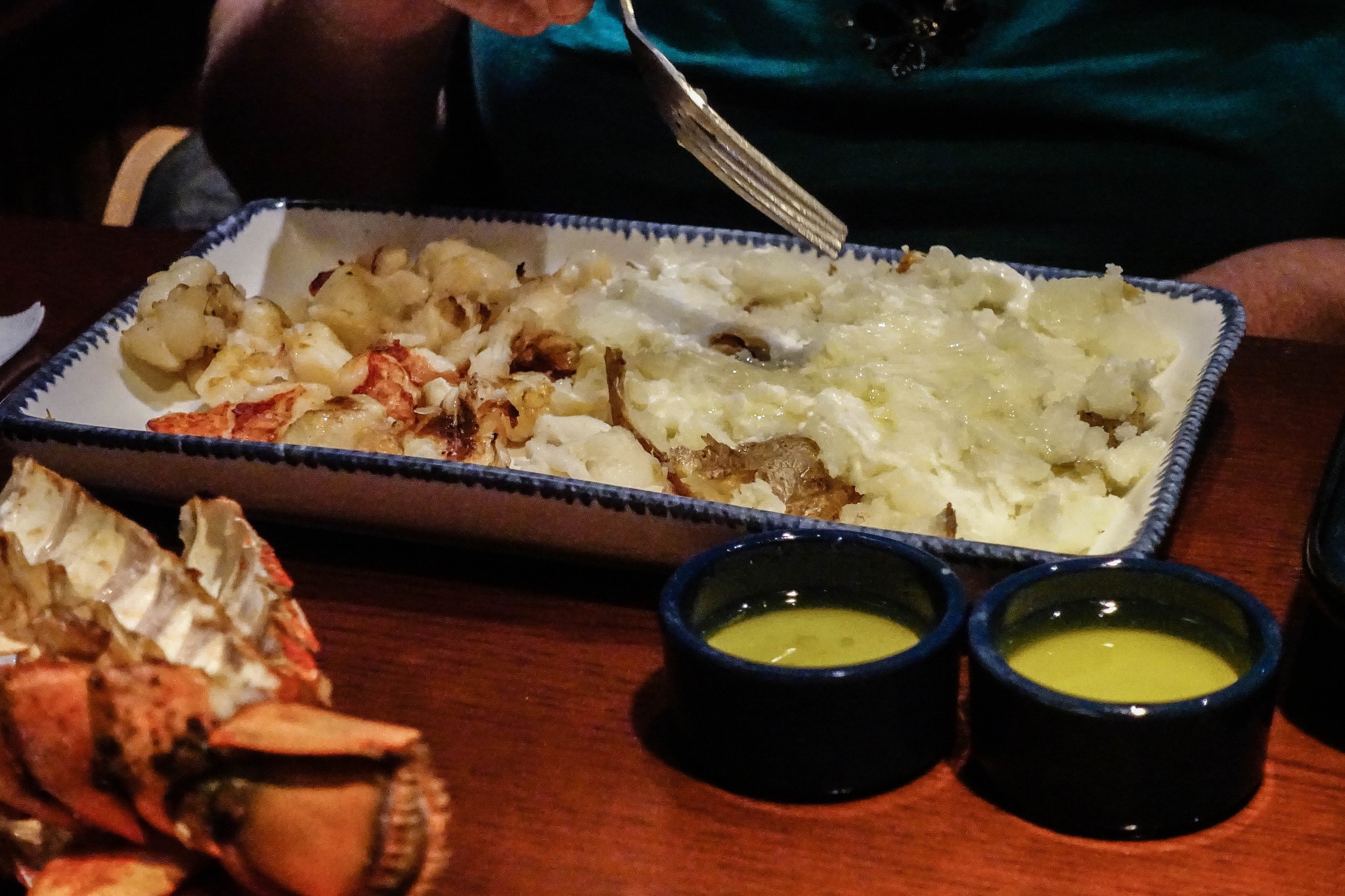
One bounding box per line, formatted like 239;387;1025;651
619;0;848;260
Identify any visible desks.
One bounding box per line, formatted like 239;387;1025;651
0;216;1344;896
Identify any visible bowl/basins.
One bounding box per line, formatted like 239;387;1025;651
966;555;1285;839
658;529;967;803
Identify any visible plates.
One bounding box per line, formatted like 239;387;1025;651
0;202;1245;584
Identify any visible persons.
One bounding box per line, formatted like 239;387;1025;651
198;0;1345;343
